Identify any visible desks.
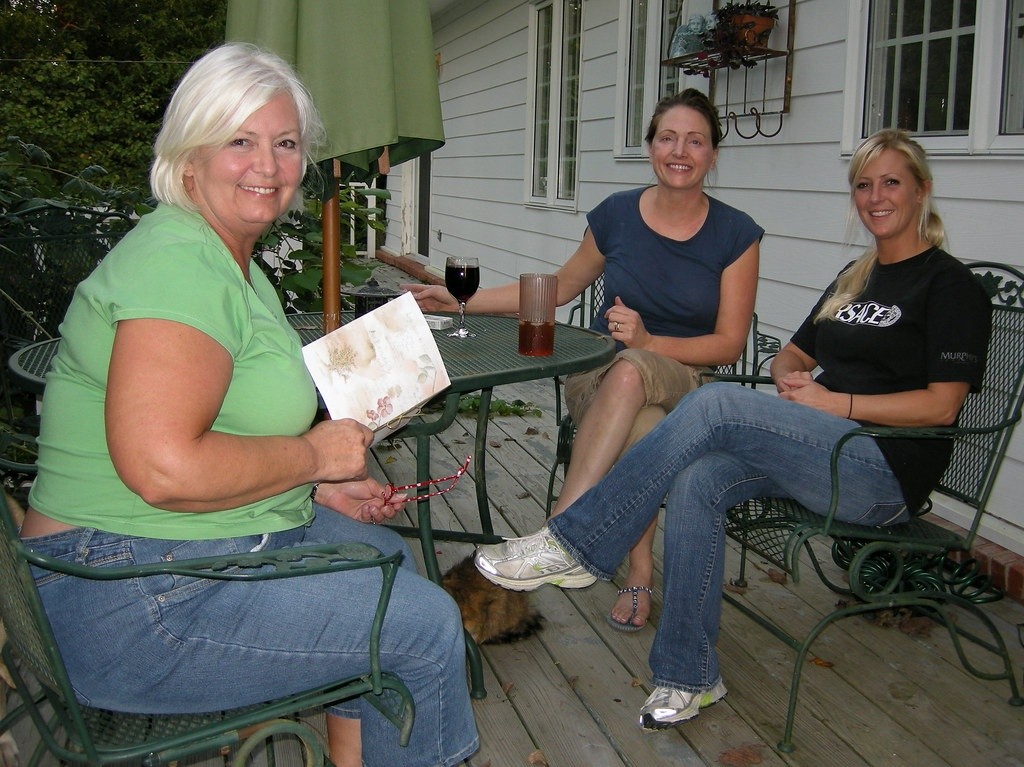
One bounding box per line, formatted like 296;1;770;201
8;305;617;588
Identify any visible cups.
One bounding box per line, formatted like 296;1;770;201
518;273;557;356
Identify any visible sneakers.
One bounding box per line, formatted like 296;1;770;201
473;525;598;592
640;671;728;731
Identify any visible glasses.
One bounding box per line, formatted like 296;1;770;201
381;455;471;505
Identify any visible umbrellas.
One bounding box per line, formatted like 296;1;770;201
226;0;446;337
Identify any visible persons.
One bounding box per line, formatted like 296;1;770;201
474;128;992;731
18;40;484;766
400;88;766;633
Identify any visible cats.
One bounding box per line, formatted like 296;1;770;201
440;549;545;647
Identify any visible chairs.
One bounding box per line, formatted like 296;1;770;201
0;475;416;767
546;224;782;584
698;260;1023;752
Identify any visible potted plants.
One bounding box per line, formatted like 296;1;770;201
710;0;781;51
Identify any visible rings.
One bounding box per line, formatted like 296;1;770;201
614;322;620;332
371;518;378;525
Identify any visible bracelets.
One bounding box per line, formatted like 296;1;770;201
847;393;852;419
310;482;320;502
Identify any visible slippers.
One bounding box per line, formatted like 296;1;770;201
607;585;652;631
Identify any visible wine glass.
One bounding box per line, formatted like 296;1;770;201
444;256;480;339
